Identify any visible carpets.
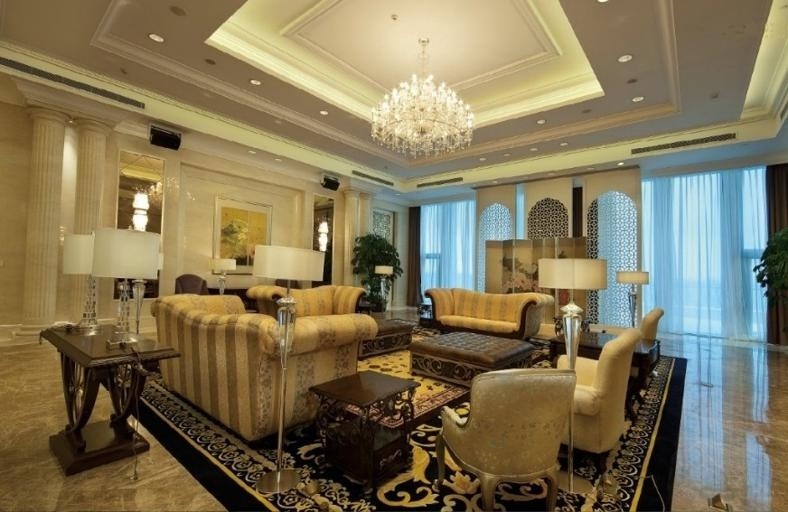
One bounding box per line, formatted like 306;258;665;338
116;319;688;511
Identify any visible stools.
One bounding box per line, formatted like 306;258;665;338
410;331;535;387
358;318;415;358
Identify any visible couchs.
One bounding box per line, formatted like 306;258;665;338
151;292;379;444
424;288;555;340
247;284;367;319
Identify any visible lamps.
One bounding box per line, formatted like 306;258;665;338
62;232;98;328
375;265;394;313
318;221;328;252
252;244;325;495
211;258;236;294
132;188;149;232
616;271;649;328
538;258;606;496
369;37;473;159
90;226;161;351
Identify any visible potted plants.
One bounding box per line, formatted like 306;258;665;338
350;232;404;314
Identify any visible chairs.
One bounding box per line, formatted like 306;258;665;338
556;328;643;491
626;310;664;378
437;369;578;511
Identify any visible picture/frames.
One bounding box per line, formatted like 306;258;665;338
212;194;272;274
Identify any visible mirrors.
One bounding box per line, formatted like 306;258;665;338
312;193;334;287
112;149;166;300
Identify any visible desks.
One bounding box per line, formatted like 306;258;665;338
40;323;181;478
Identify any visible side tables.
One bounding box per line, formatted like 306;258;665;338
308;370;421;483
548;331;660;425
